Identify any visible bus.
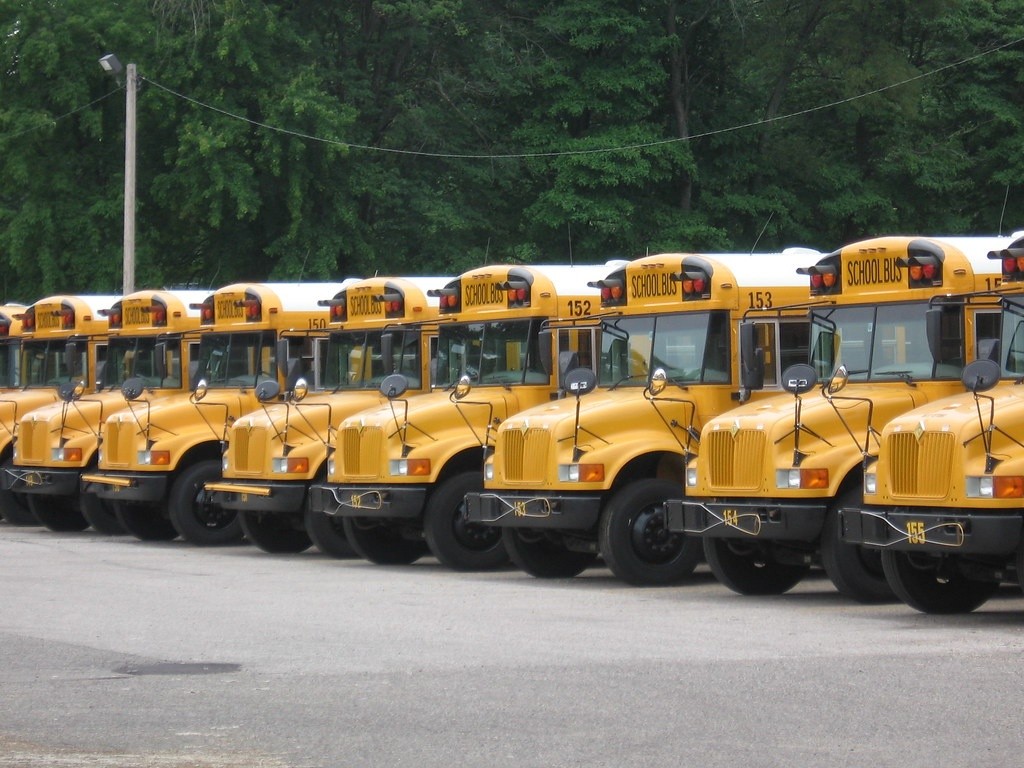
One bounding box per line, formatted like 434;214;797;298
449;245;924;589
640;230;1024;607
820;237;1024;616
287;258;707;568
0;258;464;561
